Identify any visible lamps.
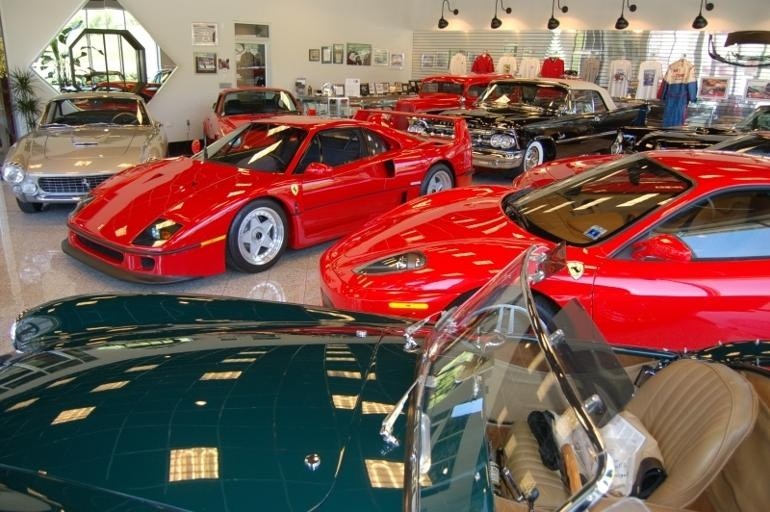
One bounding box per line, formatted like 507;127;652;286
490;0;512;29
615;0;637;30
692;0;716;28
545;0;570;31
437;0;459;29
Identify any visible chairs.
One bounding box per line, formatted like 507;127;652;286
497;351;764;511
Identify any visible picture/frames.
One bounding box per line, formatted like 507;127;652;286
333;43;344;65
420;46;476;72
346;42;372;66
374;48;388;66
742;78;770;103
321;46;332;65
328;77;423;98
695;75;731;101
190;21;220;48
389;53;405;69
308;48;321;62
195;52;218;73
